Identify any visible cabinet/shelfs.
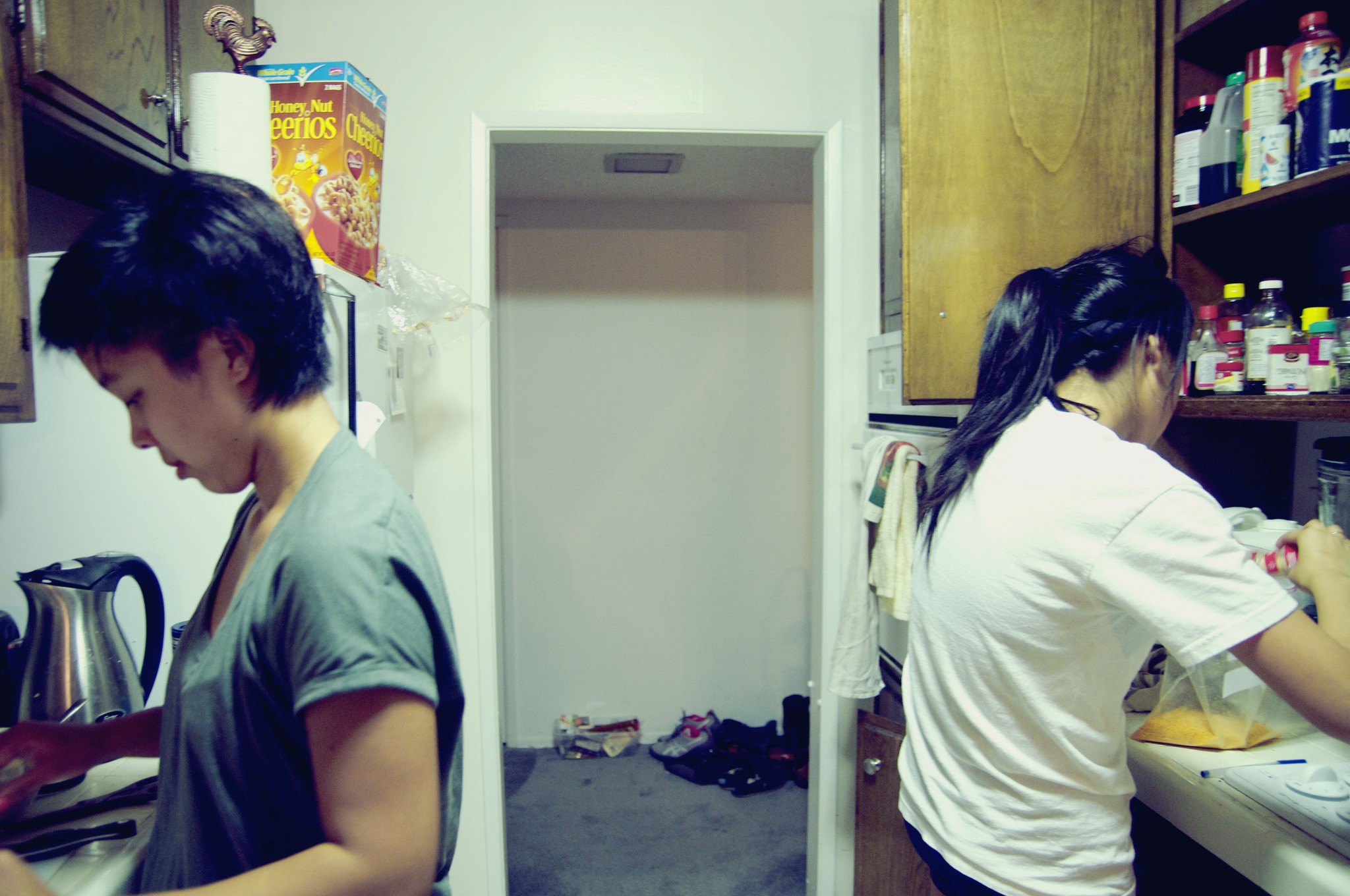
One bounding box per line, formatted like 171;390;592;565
899;3;1350;432
0;0;258;428
851;672;942;896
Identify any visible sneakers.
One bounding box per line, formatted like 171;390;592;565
717;734;809;796
649;724;713;762
663;719;780;785
657;709;721;743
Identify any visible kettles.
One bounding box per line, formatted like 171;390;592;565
12;550;165;763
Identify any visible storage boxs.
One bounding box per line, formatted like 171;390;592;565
249;62;389;284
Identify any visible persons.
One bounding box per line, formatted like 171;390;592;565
0;170;463;895
900;240;1350;896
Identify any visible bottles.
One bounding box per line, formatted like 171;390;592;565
1191;6;1342;395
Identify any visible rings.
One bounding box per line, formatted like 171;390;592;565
1332;530;1345;535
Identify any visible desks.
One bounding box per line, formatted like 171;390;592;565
1120;714;1348;896
0;774;163;896
